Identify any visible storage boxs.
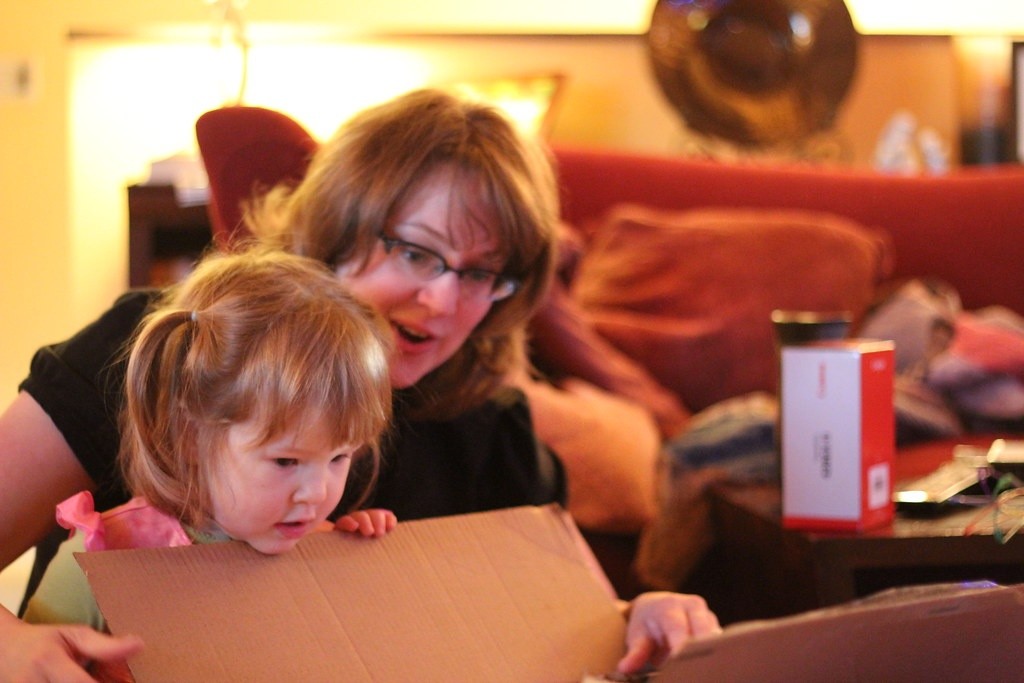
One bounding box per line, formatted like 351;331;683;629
72;500;1024;683
780;338;895;529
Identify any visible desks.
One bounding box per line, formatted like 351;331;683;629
707;433;1024;624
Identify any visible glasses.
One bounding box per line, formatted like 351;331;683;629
378;232;520;304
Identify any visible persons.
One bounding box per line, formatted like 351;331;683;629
0;90;724;683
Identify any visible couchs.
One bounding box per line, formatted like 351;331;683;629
508;138;1024;600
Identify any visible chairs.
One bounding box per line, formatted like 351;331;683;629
197;105;331;261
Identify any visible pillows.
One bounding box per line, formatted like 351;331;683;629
565;204;883;411
